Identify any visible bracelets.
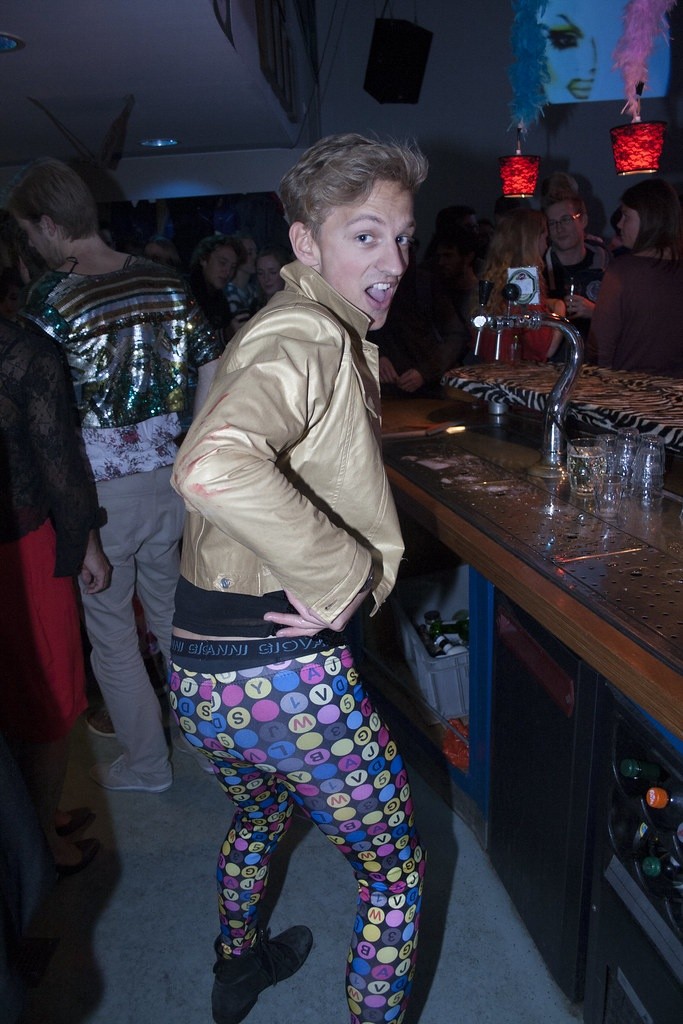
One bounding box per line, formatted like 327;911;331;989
358;562;376;594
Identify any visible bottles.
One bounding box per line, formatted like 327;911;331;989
564;277;579;317
677;822;683;844
645;786;682;817
619;758;675;789
642;850;683;893
419;623;431;652
436;635;468;656
440;620;469;634
424;610;440;630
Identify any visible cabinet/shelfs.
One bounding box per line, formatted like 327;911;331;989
352;502;683;1024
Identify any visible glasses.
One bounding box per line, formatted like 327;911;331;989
546;211;583;228
462;223;480;233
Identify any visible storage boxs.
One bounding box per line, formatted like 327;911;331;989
390;562;470;725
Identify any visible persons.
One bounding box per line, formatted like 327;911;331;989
97;218;294;344
366;197;566;400
166;132;429;1024
0;159;225;791
541;171;613;335
585;179;683;372
1;316;112;874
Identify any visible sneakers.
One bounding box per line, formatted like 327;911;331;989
84;711;119;737
89;754;173;793
172;730;216;775
145;651;169;695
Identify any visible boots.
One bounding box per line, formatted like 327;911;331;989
211;923;313;1024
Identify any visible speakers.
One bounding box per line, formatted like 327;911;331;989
363;18;434;105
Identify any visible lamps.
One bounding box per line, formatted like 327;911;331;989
496;123;541;199
610;80;667;176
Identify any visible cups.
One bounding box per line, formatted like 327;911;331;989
591;475;627;518
596;428;666;501
567;437;607;495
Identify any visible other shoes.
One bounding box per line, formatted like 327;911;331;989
49;838;99;883
53;805;92;836
